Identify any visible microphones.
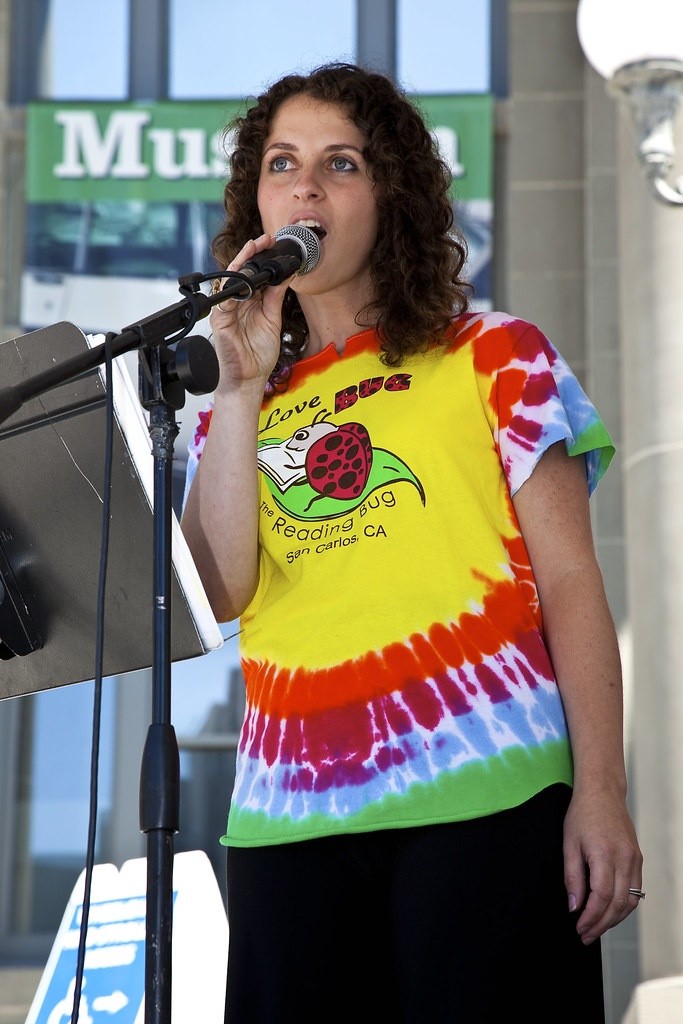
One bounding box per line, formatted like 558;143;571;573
221;225;322;296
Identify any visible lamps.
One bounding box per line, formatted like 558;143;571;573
576;0;683;204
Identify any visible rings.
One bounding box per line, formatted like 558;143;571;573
209;279;238;312
629;888;647;900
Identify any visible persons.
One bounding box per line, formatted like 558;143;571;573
177;62;649;1024
